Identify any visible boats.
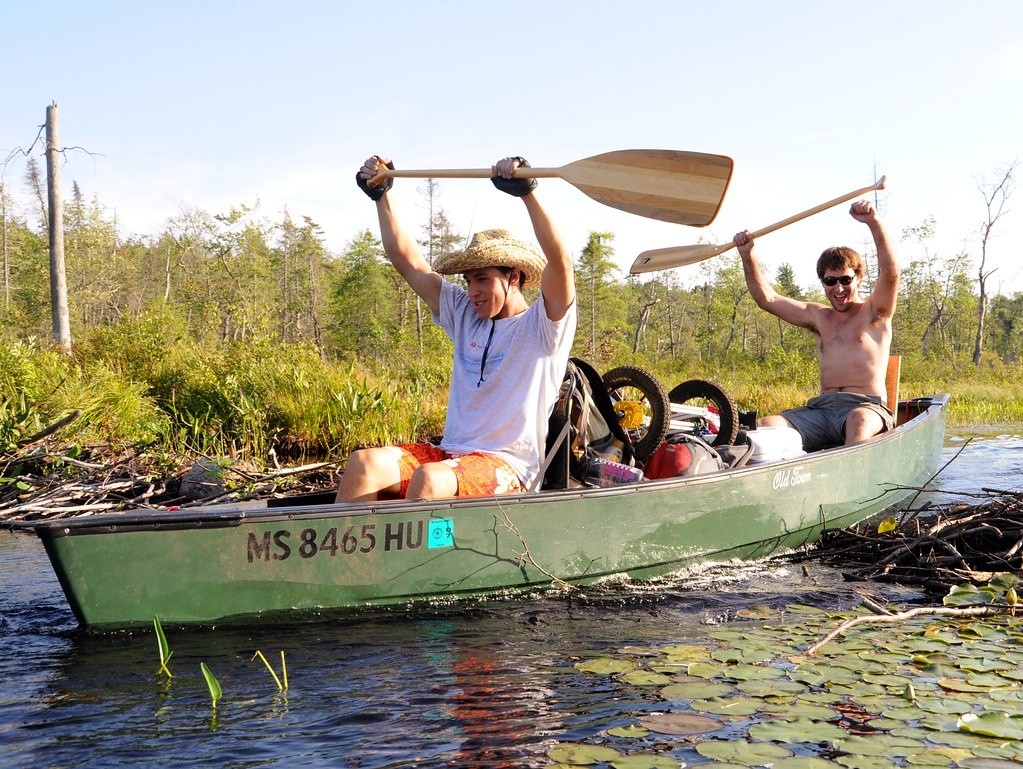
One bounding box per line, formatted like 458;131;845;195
34;355;953;637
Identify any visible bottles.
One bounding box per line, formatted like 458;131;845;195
588;457;644;483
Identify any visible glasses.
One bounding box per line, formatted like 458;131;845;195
822;274;859;287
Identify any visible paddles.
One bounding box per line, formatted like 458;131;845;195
369;147;735;229
631;174;889;274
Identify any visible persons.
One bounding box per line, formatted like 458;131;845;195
735;199;900;447
334;154;578;504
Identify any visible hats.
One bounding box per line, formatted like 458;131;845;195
432;228;548;287
